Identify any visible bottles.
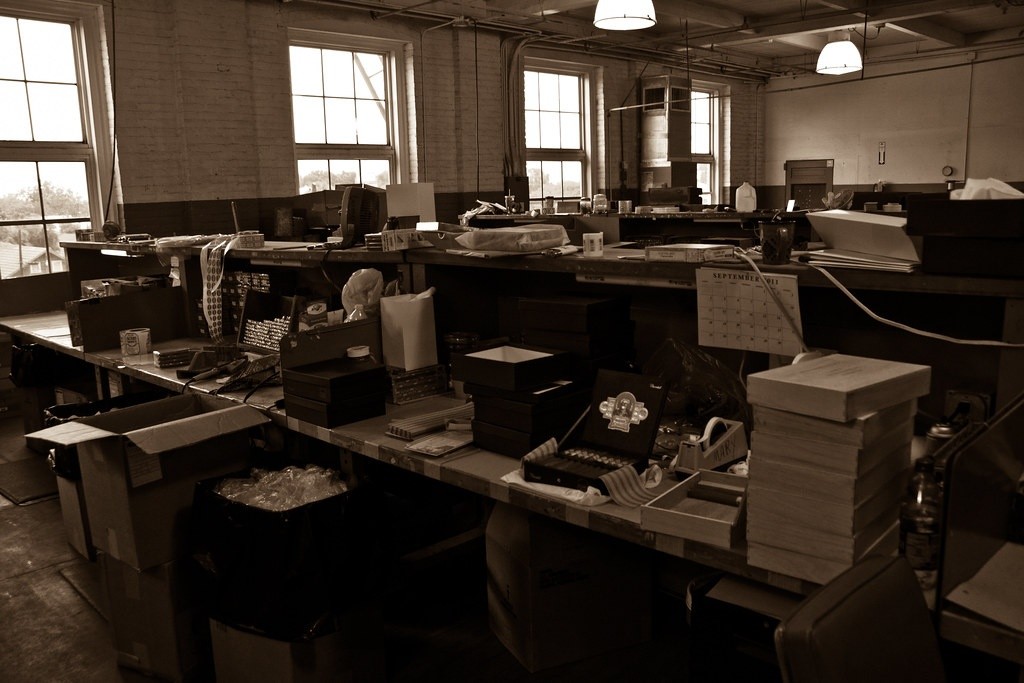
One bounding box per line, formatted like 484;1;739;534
736;181;757;213
347;304;368;322
899;458;945;582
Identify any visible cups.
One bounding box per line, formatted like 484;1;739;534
505;195;515;216
864;202;878;212
583;233;604;258
755;220;795;265
883;205;902;212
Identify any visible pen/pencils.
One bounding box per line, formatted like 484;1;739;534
231;201;240;231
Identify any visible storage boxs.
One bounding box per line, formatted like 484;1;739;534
24;344;937;683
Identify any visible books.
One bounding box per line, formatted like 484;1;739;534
806;208;921;274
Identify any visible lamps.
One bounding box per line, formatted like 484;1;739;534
816;30;863;75
593;0;658;30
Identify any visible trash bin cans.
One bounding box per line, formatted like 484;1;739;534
11;344;97;454
41;391;172;562
192;459;382;683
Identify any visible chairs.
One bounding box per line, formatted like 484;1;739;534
774;553;946;682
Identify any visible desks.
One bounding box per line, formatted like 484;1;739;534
1;215;1024;668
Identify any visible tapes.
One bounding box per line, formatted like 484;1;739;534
347;345;370;358
692;416;727;451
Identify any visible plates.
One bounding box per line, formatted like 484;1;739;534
618;200;679;214
